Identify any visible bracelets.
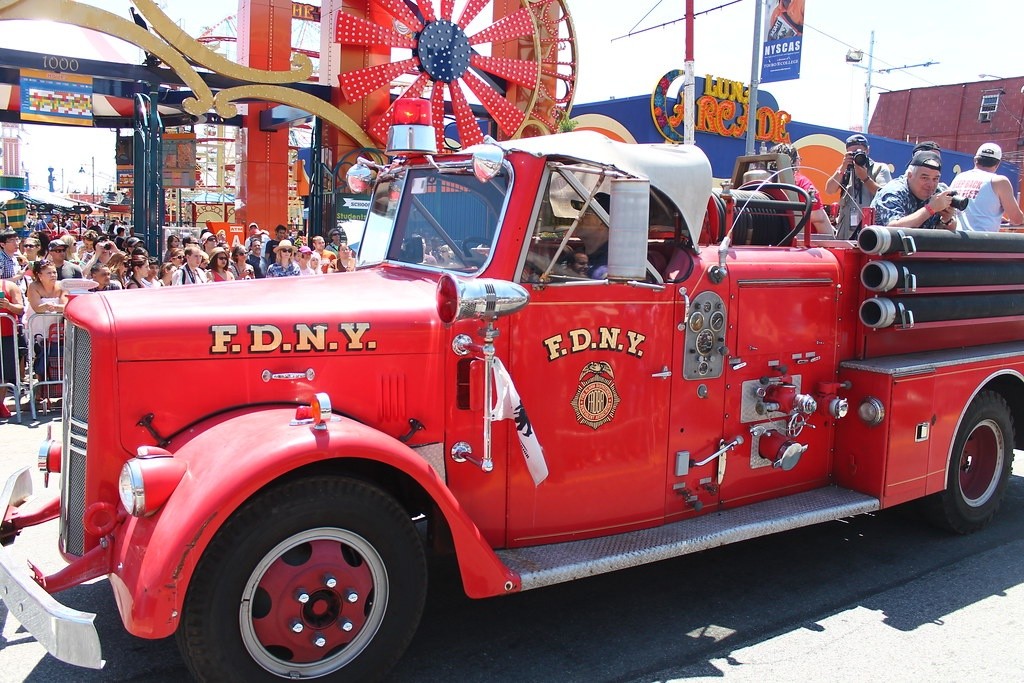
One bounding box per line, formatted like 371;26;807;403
836;167;845;175
925;204;935;216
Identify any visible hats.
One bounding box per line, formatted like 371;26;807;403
976;142;1002;160
845;135;869;148
272;240;296;253
570;192;610;214
299;246;313;254
126;236;145;247
199;232;214;245
909;151;942;171
911;140;940;157
209;247;227;261
259;229;269;236
249;222;259;229
48;239;69;250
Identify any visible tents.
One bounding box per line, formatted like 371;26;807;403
19;187;111;241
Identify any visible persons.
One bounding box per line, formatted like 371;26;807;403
525;192;610;280
566;249;589;278
767;0;805;41
0;202;461;415
825;134;891;241
768;142;834;234
869;152;957;229
905;140;941;168
950;142;1024;232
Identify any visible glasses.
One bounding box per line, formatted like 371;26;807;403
22;244;36;248
796;157;802;162
207;237;217;241
217;256;227;260
39;259;54;267
238;251;249;256
331;233;340;236
281;248;292;253
73;243;77;246
173;255;186;259
50;249;65;253
577;209;596;218
6;238;21;244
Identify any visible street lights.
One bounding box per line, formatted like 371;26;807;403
78;163;94;204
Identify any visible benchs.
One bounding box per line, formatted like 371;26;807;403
523;240;692;282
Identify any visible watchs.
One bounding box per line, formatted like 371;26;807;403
860;176;870;184
940;218;953;227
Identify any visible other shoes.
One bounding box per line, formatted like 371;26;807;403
7;382;24;394
0;403;12;418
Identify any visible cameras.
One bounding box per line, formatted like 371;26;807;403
946;190;969;211
104;244;111;250
850;149;867;166
247;270;251;275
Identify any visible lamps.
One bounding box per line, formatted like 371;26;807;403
978;74;1002;79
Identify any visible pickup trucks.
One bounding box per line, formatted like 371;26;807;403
0;129;1024;682
